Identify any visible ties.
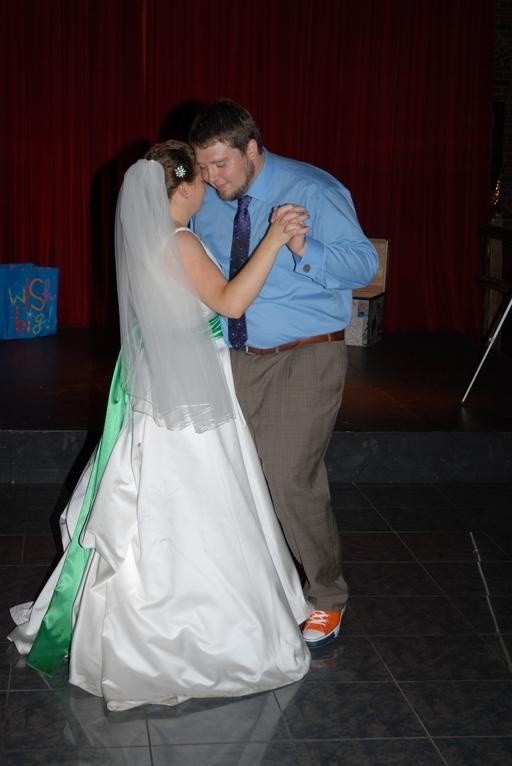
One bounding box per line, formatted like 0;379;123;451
227;194;253;350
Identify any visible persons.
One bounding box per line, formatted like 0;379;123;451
2;137;316;714
186;99;380;651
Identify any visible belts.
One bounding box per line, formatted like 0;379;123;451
246;327;346;354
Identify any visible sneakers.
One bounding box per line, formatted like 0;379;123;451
301;603;346;649
